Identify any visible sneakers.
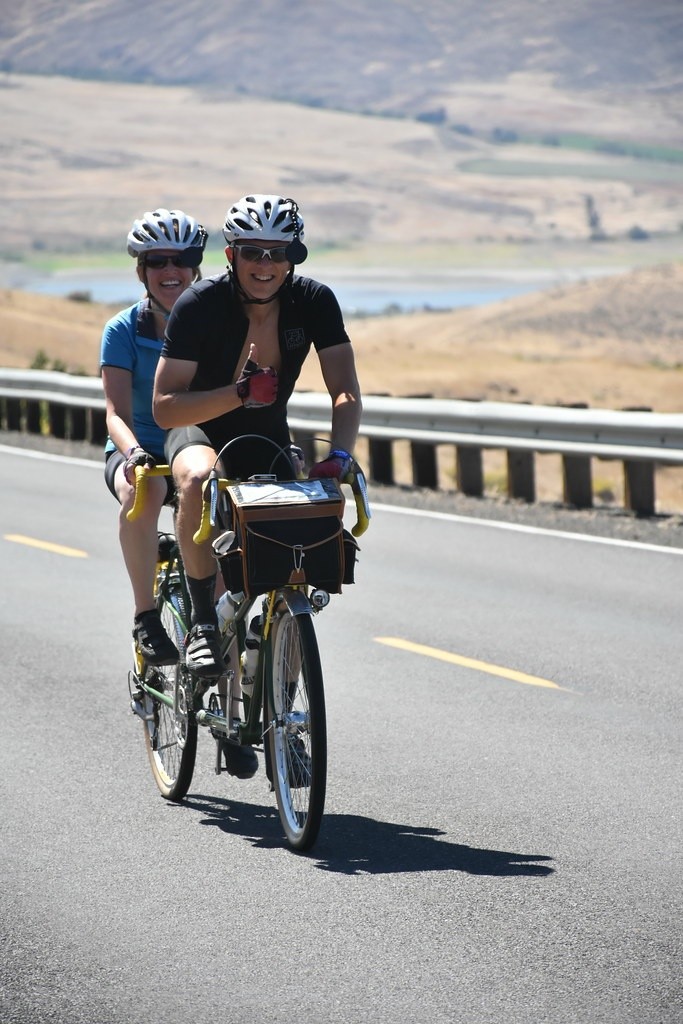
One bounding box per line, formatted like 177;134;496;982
183;624;227;678
210;719;258;780
286;734;312;788
132;609;179;665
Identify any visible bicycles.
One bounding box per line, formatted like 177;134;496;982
128;433;371;851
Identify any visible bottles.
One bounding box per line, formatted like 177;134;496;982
215;591;245;637
239;614;264;698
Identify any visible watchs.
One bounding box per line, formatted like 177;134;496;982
125;444;141;460
328;449;349;459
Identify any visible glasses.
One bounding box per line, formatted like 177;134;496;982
138;255;188;269
229;244;288;263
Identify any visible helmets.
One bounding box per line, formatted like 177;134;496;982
222;194;305;245
127;208;209;258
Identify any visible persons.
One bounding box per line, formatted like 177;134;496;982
151;196;362;791
100;209;259;778
